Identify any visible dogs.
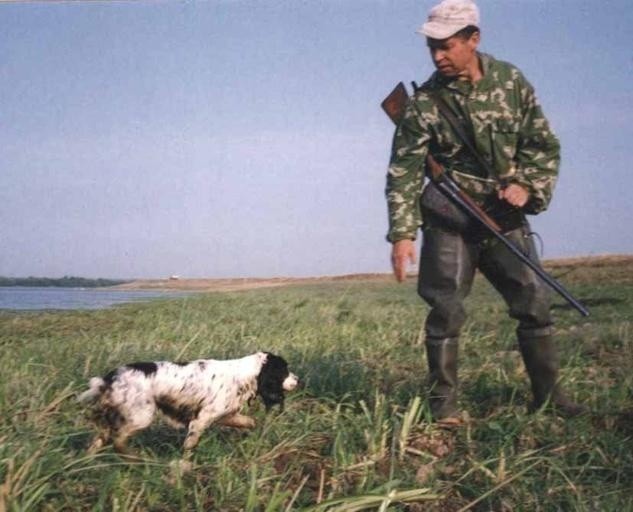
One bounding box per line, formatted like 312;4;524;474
73;351;302;476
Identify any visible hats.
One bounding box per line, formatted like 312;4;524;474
414;1;481;41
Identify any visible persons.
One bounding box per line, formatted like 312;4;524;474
384;0;587;428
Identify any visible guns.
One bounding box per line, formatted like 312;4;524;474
381;82;591;318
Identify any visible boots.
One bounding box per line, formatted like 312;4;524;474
425;338;461;425
516;325;591;418
